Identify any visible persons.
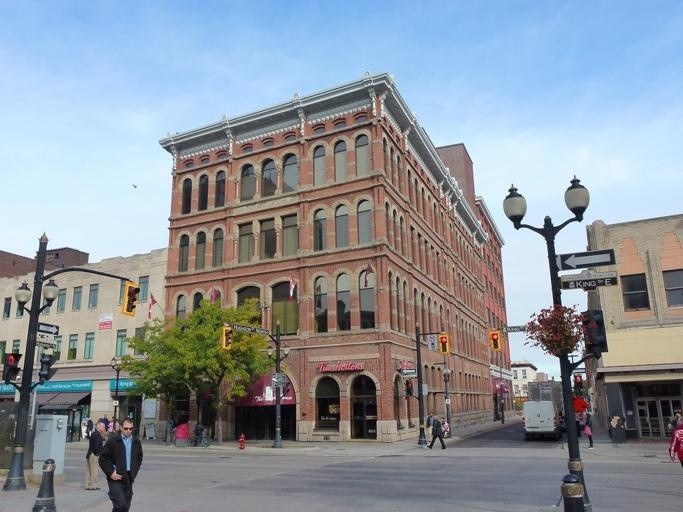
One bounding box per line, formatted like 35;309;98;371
86;413;143;512
584;413;594;449
668;411;683;467
427;415;446;449
426;412;435;427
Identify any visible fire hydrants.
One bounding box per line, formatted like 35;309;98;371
238;433;246;450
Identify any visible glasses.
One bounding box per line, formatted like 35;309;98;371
123;427;133;430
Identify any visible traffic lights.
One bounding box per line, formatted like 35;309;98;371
122;281;141;317
4;353;21;382
489;330;500;352
440;334;448;355
223;327;231;349
47;355;58;379
574;374;582;392
283;382;290;396
405;380;413;395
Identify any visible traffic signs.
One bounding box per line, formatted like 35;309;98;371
561;270;617;290
234;326;252;333
254;328;269;335
272;373;285;388
501;325;525;333
35;338;57;351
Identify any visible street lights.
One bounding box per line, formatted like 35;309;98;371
266;343;291;448
3;277;59;491
110;355;129;432
503;173;589;511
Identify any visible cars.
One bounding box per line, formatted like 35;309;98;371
557;406;587;434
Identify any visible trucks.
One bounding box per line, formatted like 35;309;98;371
520;399;562;440
